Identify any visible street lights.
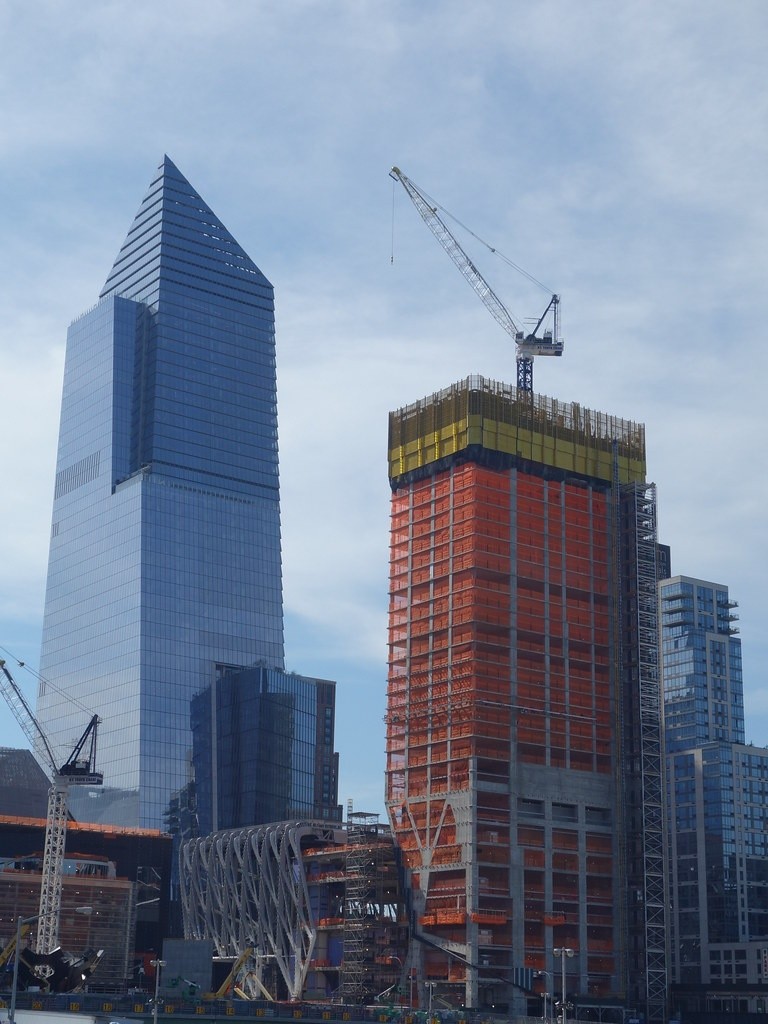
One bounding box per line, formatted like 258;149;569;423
553;946;573;1024
388;956;403;1024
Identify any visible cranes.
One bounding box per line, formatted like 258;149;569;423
0;644;108;993
388;162;566;405
